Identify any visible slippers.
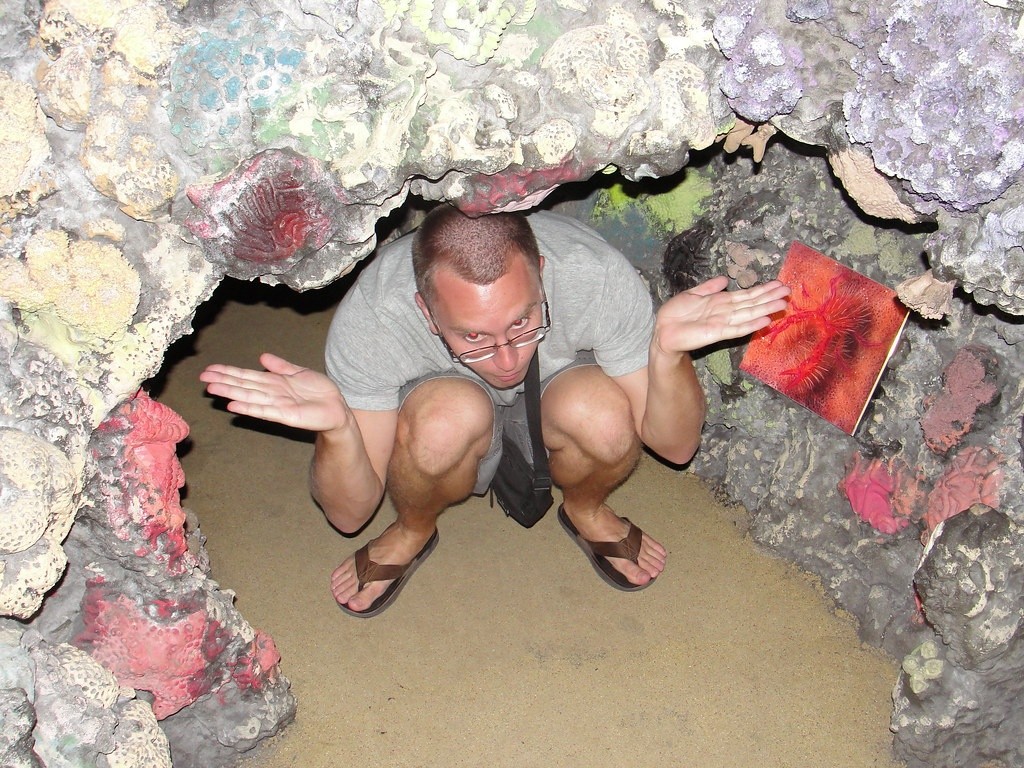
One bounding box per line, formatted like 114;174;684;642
557;503;657;591
336;526;439;618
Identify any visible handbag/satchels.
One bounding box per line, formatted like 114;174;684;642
490;432;555;528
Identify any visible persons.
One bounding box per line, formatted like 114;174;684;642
199;203;792;619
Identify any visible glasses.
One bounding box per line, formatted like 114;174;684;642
427;275;551;364
